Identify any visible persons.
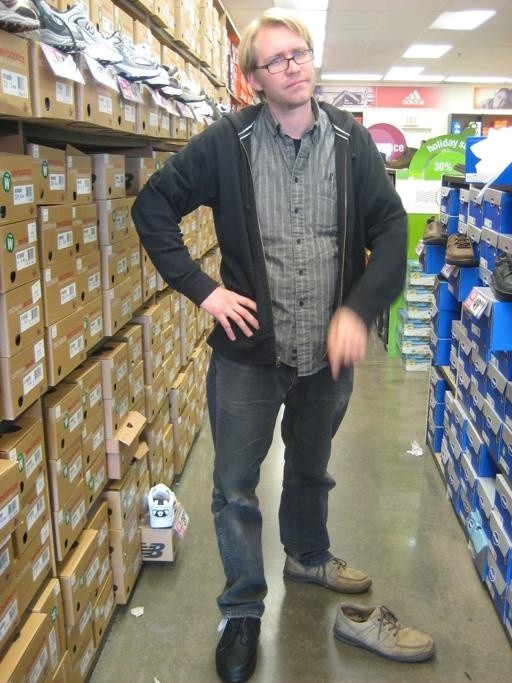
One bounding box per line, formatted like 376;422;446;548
129;8;411;682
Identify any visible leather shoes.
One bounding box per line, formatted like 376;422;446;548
282;551;372;595
215;616;261;683
423;215;450;247
383;147;418;169
489;250;512;302
454;161;464;175
444;231;478;268
332;602;434;663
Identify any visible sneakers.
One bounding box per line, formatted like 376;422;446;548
14;0;88;54
41;0;124;65
133;57;172;90
0;0;42;30
193;94;234;121
146;482;179;529
93;19;161;80
160;62;185;99
177;71;207;105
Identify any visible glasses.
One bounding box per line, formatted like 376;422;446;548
252;48;314;74
